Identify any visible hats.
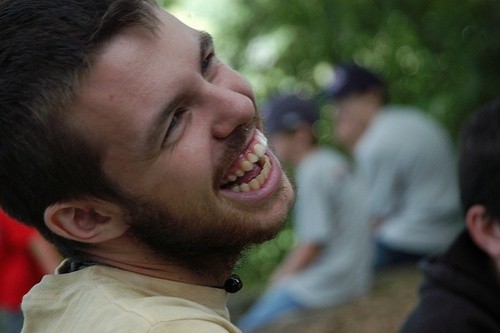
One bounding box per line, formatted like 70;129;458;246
324;64;384;97
263;93;321;132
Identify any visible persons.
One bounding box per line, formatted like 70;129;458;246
250;57;500;333
1;2;296;333
